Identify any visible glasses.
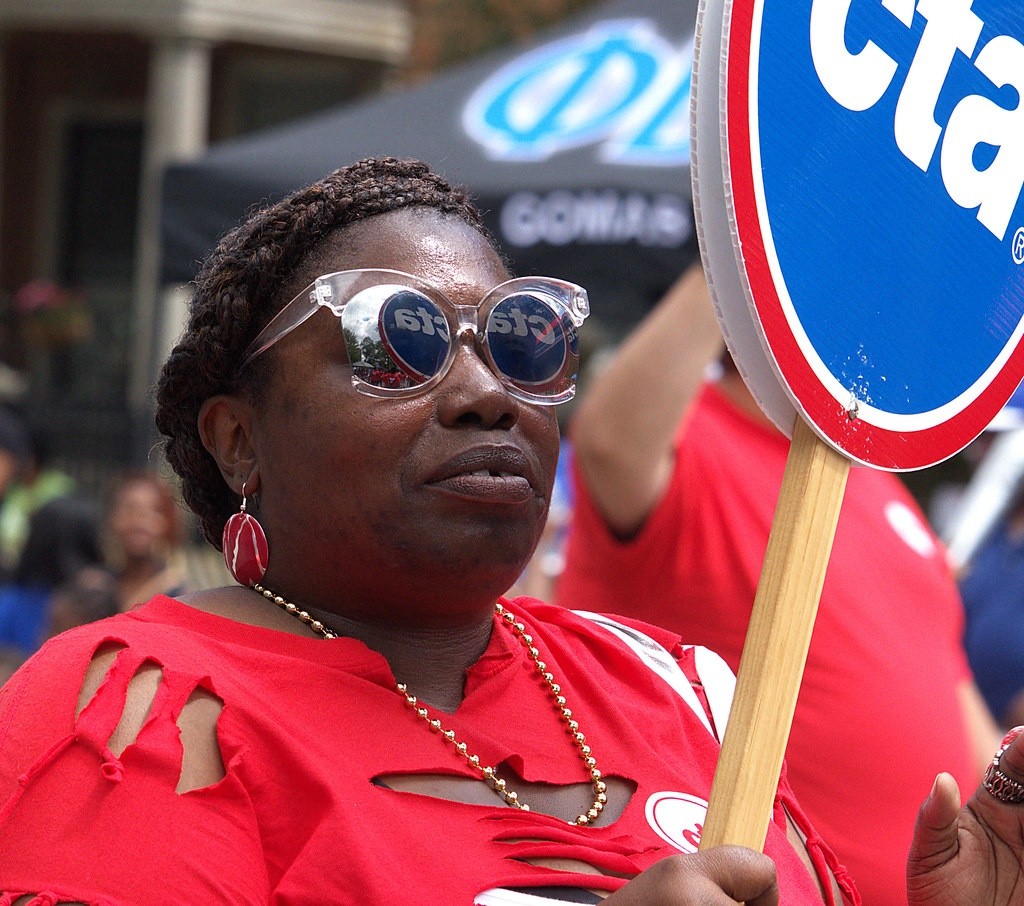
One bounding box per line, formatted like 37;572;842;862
243;269;588;405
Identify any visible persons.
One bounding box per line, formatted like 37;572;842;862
2;156;1024;906
